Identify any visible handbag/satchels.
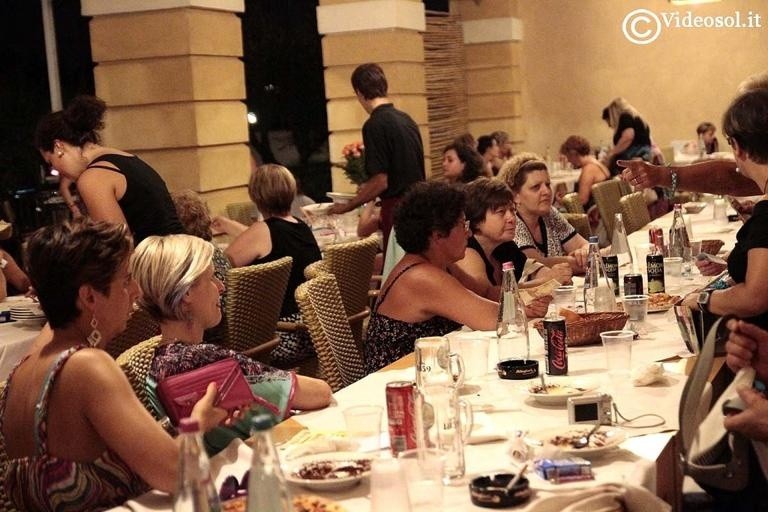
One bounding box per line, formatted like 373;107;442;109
674;310;758;494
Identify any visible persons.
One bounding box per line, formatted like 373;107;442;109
441;72;768;177
327;63;427;266
0;176;768;512
33;95;184;254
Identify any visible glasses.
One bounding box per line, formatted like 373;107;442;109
457;219;471;233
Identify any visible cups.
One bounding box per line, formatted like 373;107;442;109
600;328;636;377
340;333;492;512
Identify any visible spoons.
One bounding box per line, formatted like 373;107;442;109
569;411;614;449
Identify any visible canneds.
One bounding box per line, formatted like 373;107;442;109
624;273;643;297
601;254;619;295
646;253;665;294
542;316;569;375
385;380;418;457
648;228;664;247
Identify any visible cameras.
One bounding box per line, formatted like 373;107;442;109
568;393;613;426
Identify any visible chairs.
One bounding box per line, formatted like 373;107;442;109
0;146;704;432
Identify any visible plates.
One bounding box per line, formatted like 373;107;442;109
7;295;47;329
280;450;378;492
523;421;627;461
301;191;356;218
518;377;601;407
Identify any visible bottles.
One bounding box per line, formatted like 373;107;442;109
696;133;708;162
542;145;574;181
548;190;731;334
171;417;222;512
244;415;289;512
495;260;530;362
